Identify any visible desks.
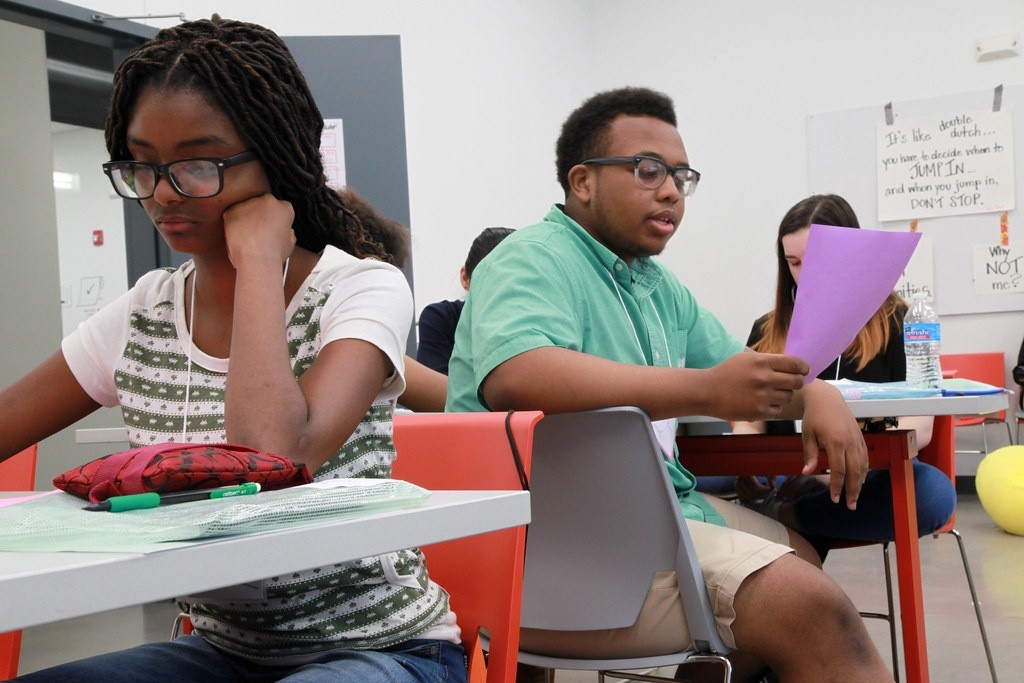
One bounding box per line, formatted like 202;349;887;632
0;488;531;635
75;392;1011;683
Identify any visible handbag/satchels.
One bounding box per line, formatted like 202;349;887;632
939;378;1015;395
52;443;314;503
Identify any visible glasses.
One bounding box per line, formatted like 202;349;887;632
581;155;700;197
102;150;258;200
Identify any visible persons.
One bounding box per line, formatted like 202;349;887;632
443;89;899;683
1;16;467;683
697;193;955;563
351;209;519;414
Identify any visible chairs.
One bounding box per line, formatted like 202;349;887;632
182;410;545;683
858;369;999;683
478;407;734;683
941;351;1014;456
0;441;39;683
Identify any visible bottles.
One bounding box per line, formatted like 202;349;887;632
902;293;943;389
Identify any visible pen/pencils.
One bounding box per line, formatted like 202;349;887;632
80;482;262;514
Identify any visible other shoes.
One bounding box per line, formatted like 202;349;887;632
729;496;755;510
